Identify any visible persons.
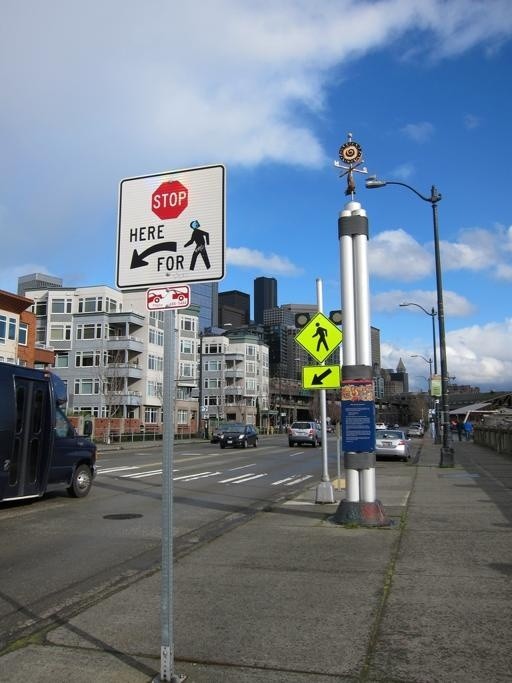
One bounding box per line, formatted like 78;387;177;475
464;420;472;442
456;419;464;441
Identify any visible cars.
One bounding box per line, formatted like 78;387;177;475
376;423;386;430
211;423;258;448
406;423;424;437
375;430;412;462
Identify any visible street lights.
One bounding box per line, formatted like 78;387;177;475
411;355;432;409
400;303;441;444
366;180;456;468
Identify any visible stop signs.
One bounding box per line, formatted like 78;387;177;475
115;163;226;291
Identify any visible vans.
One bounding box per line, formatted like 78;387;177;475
0;362;97;503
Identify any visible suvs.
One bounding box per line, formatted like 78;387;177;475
288;422;321;448
